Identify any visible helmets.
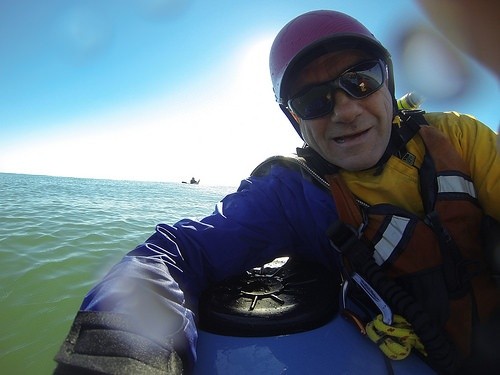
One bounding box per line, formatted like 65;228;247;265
269;11;389;102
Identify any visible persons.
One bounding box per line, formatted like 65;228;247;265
57;0;498;373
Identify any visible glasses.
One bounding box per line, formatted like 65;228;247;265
282;58;386;119
